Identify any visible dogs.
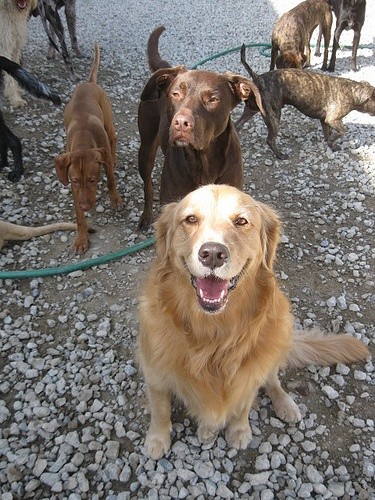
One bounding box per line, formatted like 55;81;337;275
136;185;369;461
0;0;375;255
